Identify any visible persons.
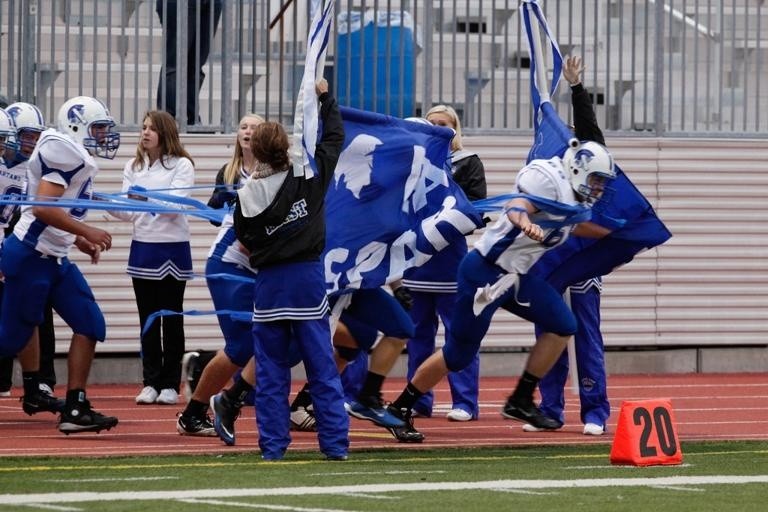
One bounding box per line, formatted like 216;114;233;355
231;77;350;460
154;0;222;126
1;53;609;442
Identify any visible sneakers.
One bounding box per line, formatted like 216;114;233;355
0;383;119;433
174;410;219;438
583;423;609;436
208;389;242;445
382;403;425;443
287;403;319;432
182;349;212;406
343;391;408;428
134;385;180;405
500;396;564;433
445;408;479;421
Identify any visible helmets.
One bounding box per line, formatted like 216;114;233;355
57;95;115;146
0;107;18;137
4;102;47;133
561;140;616;197
